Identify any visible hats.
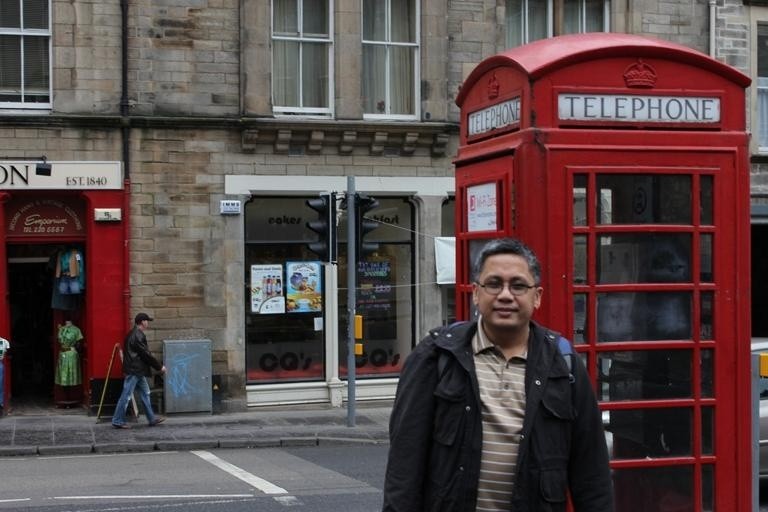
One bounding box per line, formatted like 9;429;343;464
135;313;153;323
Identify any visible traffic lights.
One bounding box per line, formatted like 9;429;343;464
307;194;332;265
355;192;380;263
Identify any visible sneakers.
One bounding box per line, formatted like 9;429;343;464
112;422;130;429
657;426;671;454
149;415;166;425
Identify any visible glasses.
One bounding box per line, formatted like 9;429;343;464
475;281;539;295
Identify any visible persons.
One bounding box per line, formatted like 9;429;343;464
54;320;84;387
112;313;167;429
0;337;10;408
382;236;613;511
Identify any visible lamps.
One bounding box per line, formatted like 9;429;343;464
0;152;53;178
